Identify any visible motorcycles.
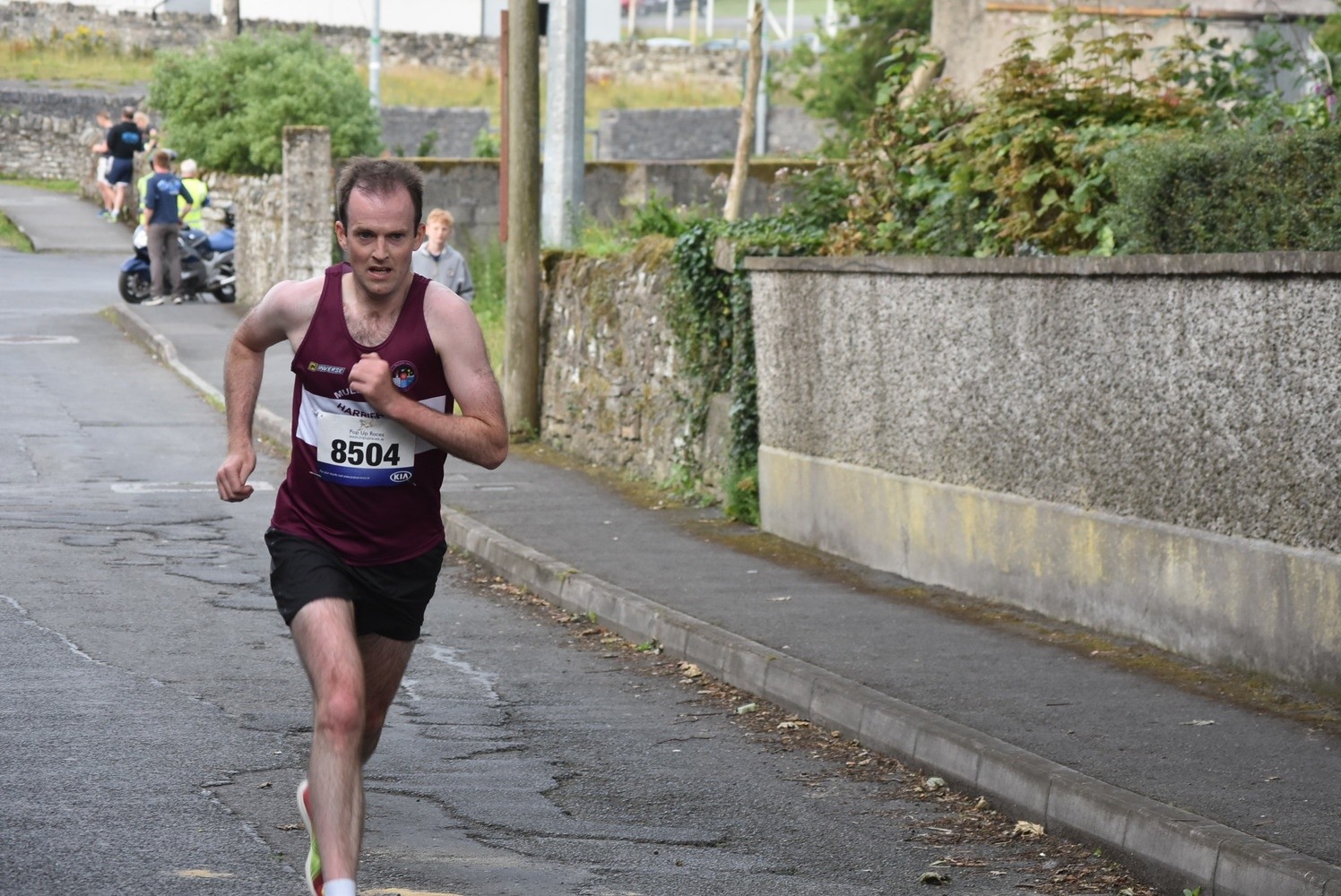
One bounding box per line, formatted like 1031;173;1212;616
117;207;235;305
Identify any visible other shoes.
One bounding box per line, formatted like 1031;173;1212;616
107;213;118;223
141;294;163;305
98;210;110;217
172;295;181;304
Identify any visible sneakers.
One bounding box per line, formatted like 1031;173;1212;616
296;780;324;896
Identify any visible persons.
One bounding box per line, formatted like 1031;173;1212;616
410;207;475;309
217;161;510;896
92;107;210;307
